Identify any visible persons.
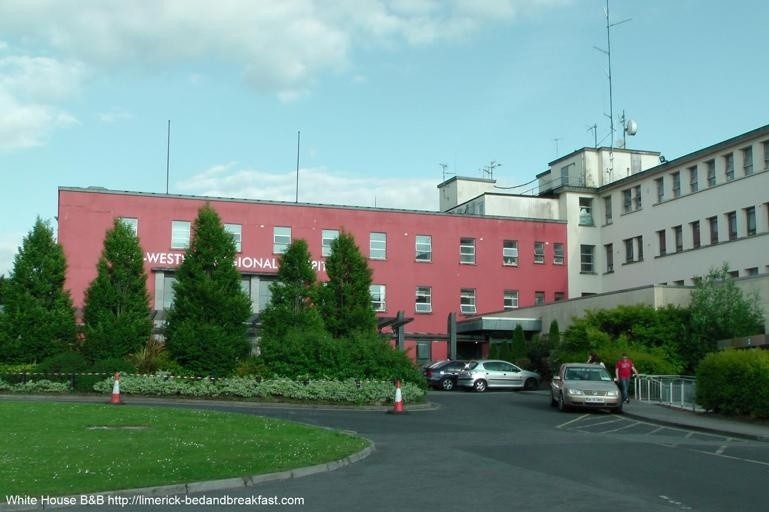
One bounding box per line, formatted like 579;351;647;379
587;351;605;367
614;352;641;404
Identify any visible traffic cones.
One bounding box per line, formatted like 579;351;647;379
109;370;123;405
386;380;408;415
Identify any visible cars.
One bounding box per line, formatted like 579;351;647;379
418;359;543;392
550;363;623;413
